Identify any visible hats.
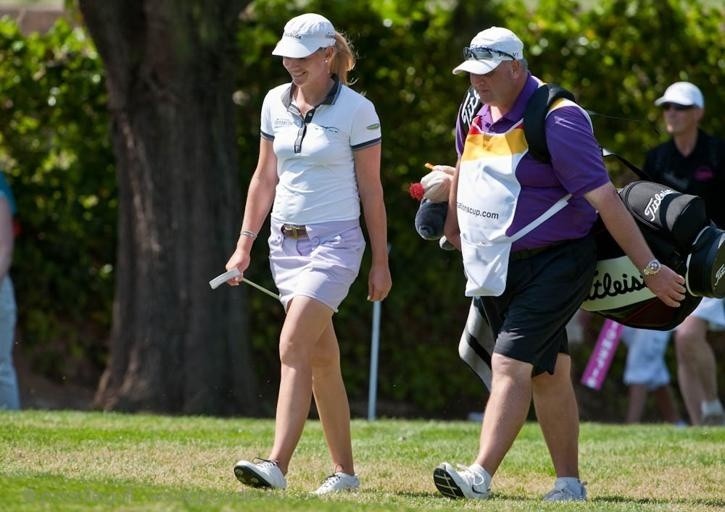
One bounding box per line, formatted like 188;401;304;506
656;81;705;107
270;12;336;60
451;25;524;75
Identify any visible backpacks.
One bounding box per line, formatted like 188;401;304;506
458;85;725;331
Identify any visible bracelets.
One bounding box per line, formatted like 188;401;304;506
239;230;257;240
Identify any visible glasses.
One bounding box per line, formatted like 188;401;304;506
662;103;692;112
462;45;516;63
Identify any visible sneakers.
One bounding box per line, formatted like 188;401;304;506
311;470;361;497
430;463;489;501
543;486;587;501
233;459;285;491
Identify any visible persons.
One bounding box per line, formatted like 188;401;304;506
0;169;21;411
621;326;687;426
225;12;394;496
433;26;687;501
638;80;725;427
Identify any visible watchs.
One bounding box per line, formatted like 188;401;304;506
639;259;661;277
